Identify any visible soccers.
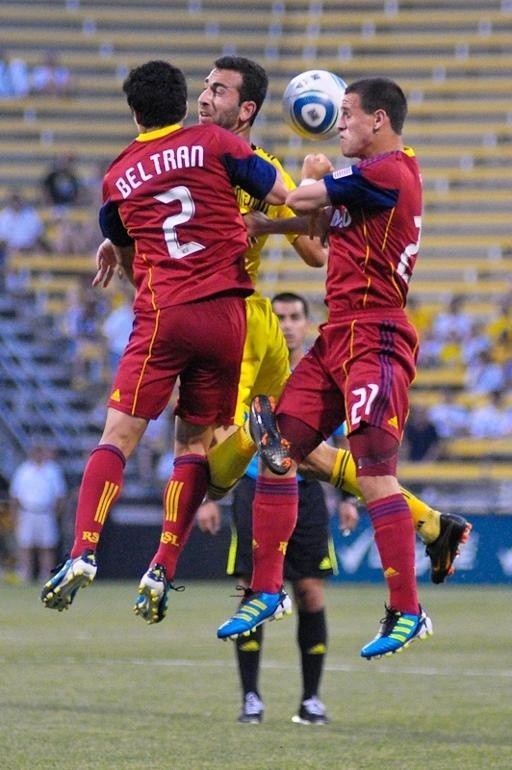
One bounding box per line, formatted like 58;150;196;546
280;68;347;141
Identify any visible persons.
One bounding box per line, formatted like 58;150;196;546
1;44;512;658
192;291;361;729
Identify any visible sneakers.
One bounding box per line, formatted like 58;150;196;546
134;563;172;623
217;585;295;642
424;512;473;585
291;694;329;726
41;549;99;612
360;602;434;661
239;689;266;725
249;395;292;475
